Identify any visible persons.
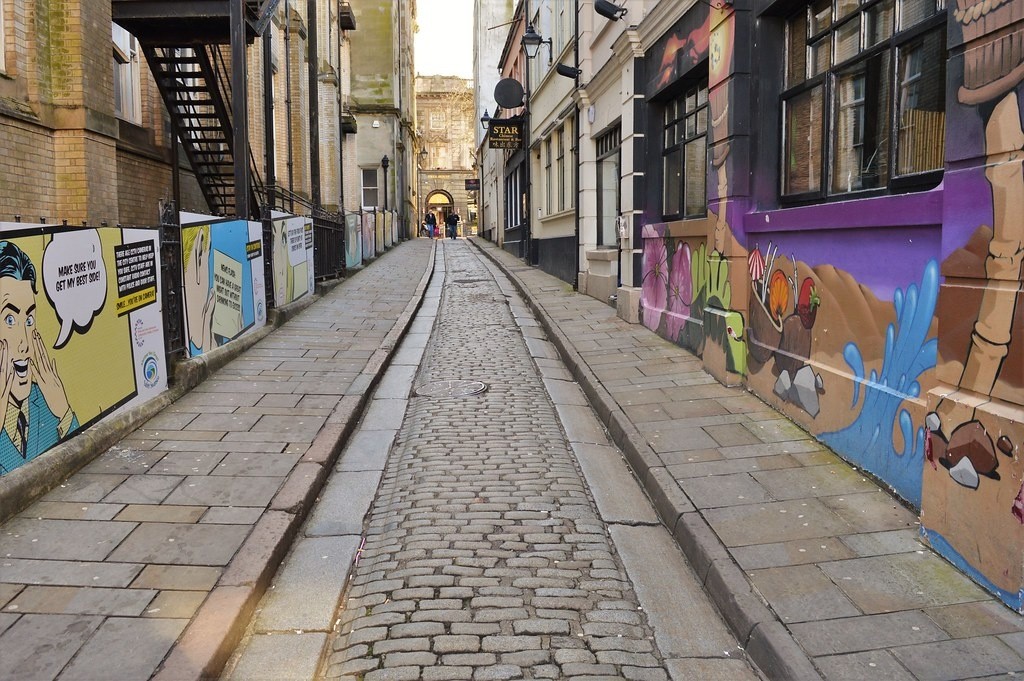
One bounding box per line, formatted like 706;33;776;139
439;221;444;238
445;212;459;240
421;221;426;236
425;210;436;239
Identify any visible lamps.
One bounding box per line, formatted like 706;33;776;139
520;19;553;62
